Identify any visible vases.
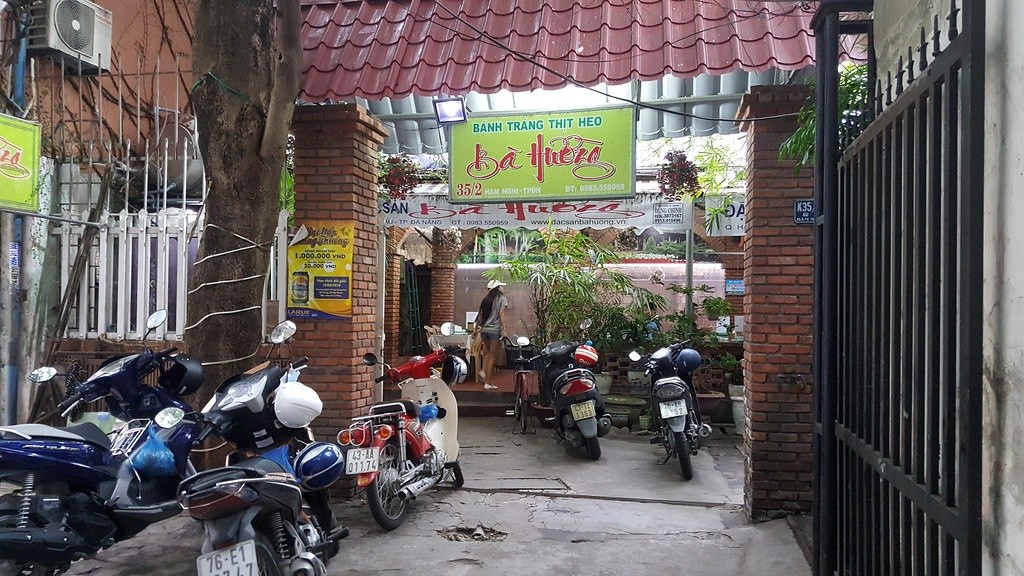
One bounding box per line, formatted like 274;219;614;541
729;395;744;436
601;396;645;428
605;410;630;428
639;416;651;429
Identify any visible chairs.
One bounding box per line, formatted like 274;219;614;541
432;324;441;332
423;325;436;339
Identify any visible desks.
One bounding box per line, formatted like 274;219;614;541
429;332;475;381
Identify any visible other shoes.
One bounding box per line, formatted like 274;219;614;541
484;383;498;389
478;370;486;382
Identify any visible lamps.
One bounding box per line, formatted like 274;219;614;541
433;93;470;122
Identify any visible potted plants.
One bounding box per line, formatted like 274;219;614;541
479;216;745;428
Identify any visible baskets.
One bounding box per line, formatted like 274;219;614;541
398;366;442;390
505;344;534;370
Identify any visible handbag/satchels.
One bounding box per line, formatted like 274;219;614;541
130;425;177;479
472;331;489;358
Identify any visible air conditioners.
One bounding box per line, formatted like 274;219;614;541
26;0;114;74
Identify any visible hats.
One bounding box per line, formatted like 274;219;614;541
487;280;506;291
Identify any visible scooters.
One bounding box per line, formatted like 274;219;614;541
0;309;204;576
154;320;351;576
530;318;612;461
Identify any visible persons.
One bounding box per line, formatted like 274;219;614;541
471;280;508;390
644;302;660;340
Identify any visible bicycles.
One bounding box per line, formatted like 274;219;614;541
500;333;544;434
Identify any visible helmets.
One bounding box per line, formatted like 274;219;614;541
575;345;599;367
293;441;345;492
273;381;323;428
676;349;701;373
158;352;204;396
441;355;467;386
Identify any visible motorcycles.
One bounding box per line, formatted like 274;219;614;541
339;322;465;530
627;339;712;481
